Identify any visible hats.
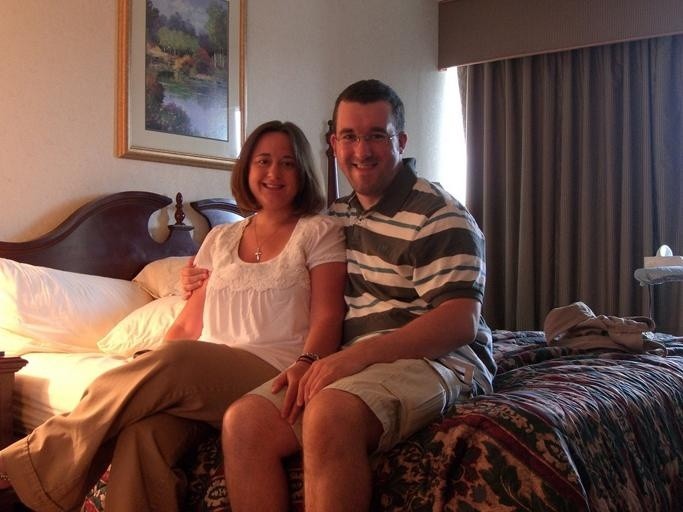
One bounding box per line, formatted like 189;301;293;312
544;300;598;346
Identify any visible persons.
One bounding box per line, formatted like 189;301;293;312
0;120;348;512
182;78;496;512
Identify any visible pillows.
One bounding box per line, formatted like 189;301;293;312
98;253;198;354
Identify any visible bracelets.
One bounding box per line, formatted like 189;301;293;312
295;352;320;365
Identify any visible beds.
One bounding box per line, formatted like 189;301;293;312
0;190;683;512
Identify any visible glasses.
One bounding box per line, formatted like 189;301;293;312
334;132;403;143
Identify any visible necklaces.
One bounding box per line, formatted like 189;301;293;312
254;213;293;262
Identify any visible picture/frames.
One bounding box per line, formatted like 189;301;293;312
113;0;250;174
